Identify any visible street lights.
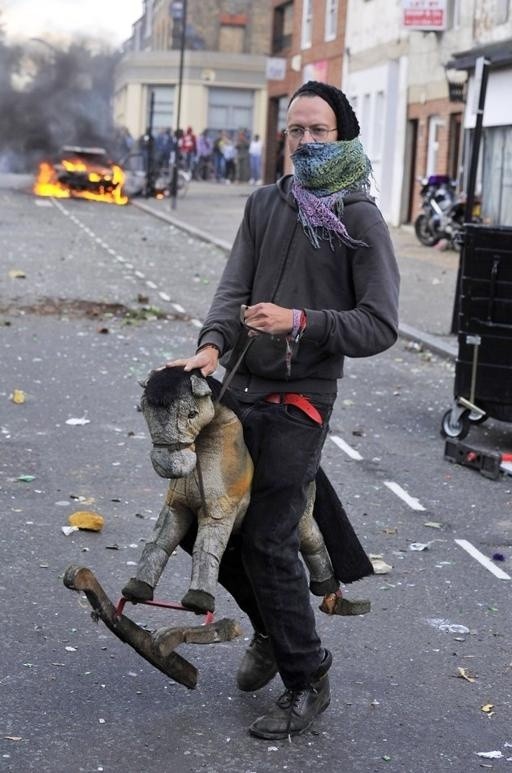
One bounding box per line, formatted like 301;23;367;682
168;0;189;203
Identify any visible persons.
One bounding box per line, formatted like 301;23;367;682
167;79;402;740
110;123;265;186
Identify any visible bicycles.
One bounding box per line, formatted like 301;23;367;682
190;157;237;183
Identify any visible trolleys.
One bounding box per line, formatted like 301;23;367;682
443;32;512;442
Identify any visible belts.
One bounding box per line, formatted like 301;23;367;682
267;393;323;427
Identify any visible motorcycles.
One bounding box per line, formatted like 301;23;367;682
413;164;486;254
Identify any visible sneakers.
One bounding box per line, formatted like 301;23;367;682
236;633;277;692
248;648;332;740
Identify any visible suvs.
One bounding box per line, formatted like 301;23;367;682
202;126;254;143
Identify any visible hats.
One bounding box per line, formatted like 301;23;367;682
288;82;360;140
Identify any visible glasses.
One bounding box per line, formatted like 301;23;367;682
285;126;336;138
